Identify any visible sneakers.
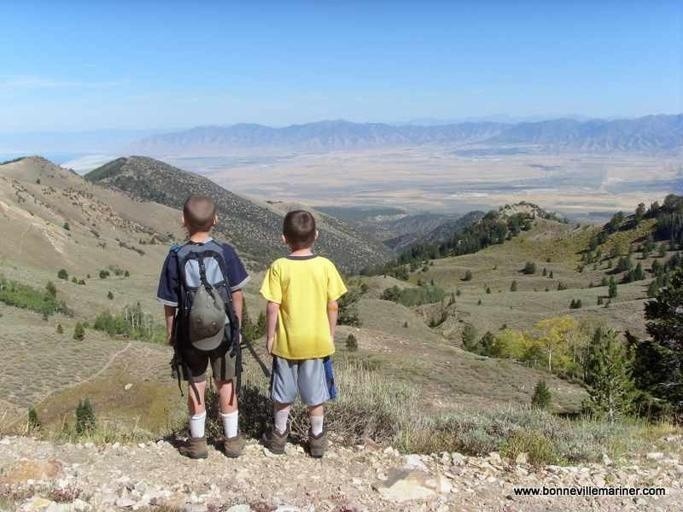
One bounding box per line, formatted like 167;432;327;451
264;431;289;454
224;430;241;457
179;436;209;459
308;427;326;457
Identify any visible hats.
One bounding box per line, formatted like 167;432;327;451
189;283;225;351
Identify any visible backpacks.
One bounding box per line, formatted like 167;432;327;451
170;239;236;357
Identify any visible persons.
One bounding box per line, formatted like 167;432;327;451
258;210;348;457
157;196;252;459
